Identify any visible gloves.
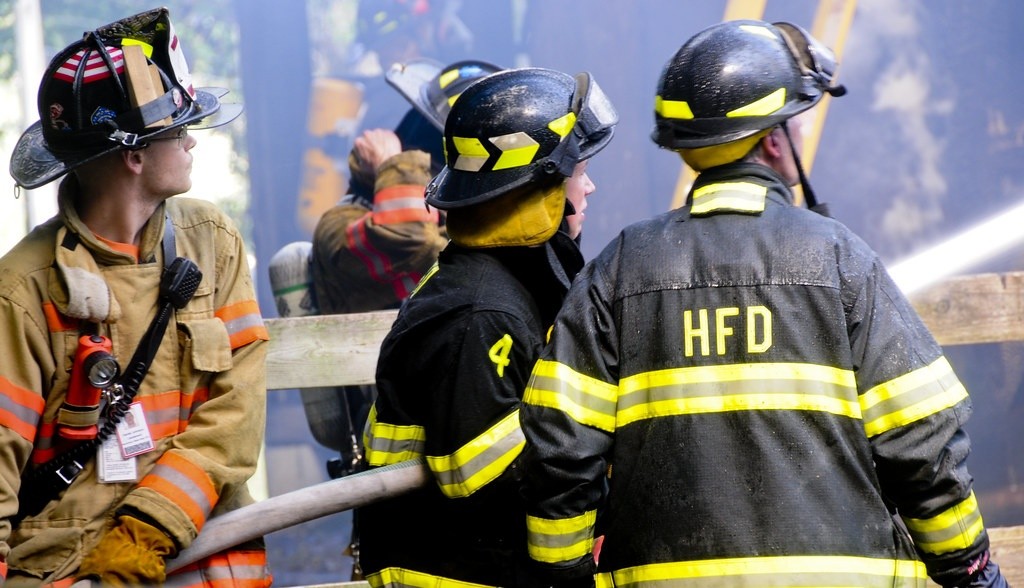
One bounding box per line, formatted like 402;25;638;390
918;539;1009;588
74;515;177;588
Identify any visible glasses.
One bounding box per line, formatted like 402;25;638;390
147;125;188;147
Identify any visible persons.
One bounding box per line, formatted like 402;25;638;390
517;19;1013;587
0;8;272;587
356;69;620;588
307;60;503;485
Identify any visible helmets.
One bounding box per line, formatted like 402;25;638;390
384;59;502;134
650;17;825;150
8;32;222;190
424;67;613;208
